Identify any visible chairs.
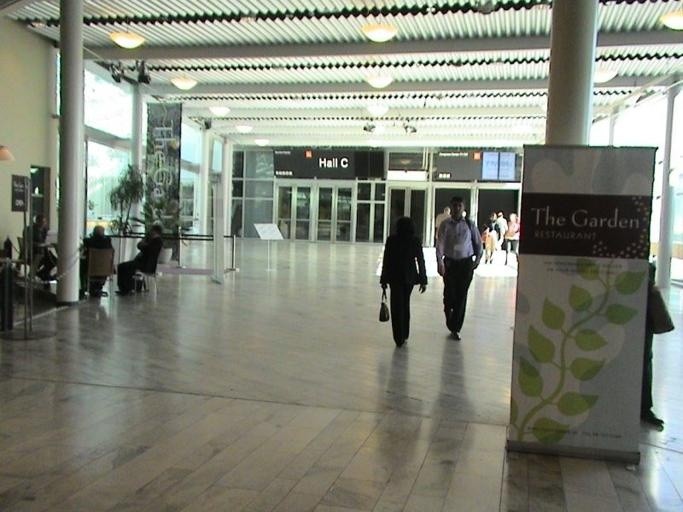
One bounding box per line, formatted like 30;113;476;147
82;248;115;300
133;248;173;298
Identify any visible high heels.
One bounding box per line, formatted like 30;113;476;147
397;338;408;347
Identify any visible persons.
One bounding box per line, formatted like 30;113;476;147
483;211;519;265
85;225;113;297
436;198;483;341
436;206;450;240
639;262;664;425
380;217;427;347
24;214;55;280
115;224;163;294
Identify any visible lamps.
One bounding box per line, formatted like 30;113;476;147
0;145;14;161
362;22;397;119
110;0;268;146
660;9;683;30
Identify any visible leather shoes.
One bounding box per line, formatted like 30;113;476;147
116;290;133;297
642;410;665;427
449;329;463;340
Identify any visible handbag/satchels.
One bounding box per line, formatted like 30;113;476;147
379;302;389;322
648;286;674;334
